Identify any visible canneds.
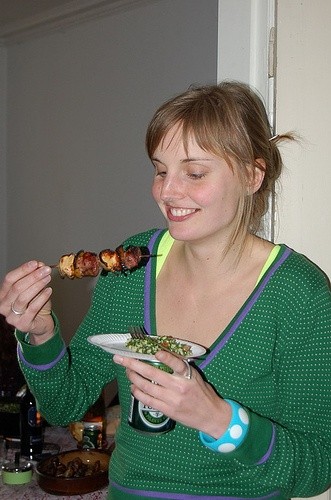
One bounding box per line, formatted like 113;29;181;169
128;360;172;435
5;438;21;458
82;427;102;449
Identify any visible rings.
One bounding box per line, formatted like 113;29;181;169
11;304;24;316
176;358;192;380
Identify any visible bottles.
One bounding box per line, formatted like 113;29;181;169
20;384;44;460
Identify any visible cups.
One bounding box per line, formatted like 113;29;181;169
42;444;60;455
2;440;33;485
5;438;21;463
77;421;103;448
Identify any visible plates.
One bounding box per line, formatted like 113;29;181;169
86;334;208;361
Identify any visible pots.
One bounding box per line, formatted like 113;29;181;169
35;443;115;495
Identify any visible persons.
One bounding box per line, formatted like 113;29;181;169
0;82;330;500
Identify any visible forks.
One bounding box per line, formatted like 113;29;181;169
128;324;193;361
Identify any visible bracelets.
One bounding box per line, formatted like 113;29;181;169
199;399;249;452
